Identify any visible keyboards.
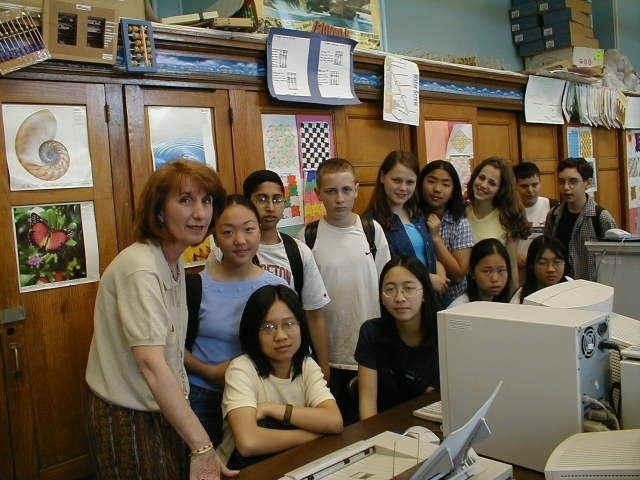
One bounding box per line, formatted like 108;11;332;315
412;400;441;422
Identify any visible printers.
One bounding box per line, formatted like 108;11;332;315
277;379;514;480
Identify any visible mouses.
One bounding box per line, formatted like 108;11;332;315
605;228;631;240
403;425;441;445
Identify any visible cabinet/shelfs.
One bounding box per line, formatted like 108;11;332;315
0;66;125;480
115;74;236;277
414;75;520;194
620;95;640;236
521;107;624;230
229;77;414;239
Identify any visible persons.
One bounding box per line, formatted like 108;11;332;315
417;159;476;301
294;158;393;426
509;236;575;304
354;253;447;421
177;193;297;479
214;283;345;471
242;169;331;390
362;150;453;307
544;156;619;282
445;237;513;310
512;160;560;290
463;156;533;303
82;157;242;480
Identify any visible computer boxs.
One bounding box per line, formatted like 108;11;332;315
436;300;609;474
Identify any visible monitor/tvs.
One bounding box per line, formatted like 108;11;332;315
521;278;640;411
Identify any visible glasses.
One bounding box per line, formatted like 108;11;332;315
382;288;422;298
262;322;298;331
253;196;285;205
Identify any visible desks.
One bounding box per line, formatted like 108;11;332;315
224;386;543;480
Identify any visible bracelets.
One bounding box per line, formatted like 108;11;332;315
191;442;215;458
283;402;293;426
